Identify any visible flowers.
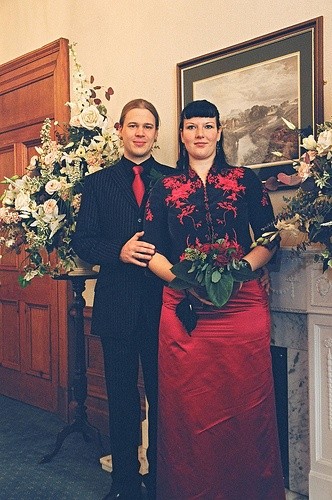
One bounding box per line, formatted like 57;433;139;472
168;232;245;336
0;43;160;286
251;120;332;274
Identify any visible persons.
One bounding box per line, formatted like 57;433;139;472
71;99;177;499
143;99;291;500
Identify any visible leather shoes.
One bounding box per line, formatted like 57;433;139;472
102;483;143;500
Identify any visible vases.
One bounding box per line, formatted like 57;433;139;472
68;250;99;276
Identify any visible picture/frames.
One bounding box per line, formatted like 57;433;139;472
174;15;323;190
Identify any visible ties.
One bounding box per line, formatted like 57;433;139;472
131;166;145;208
